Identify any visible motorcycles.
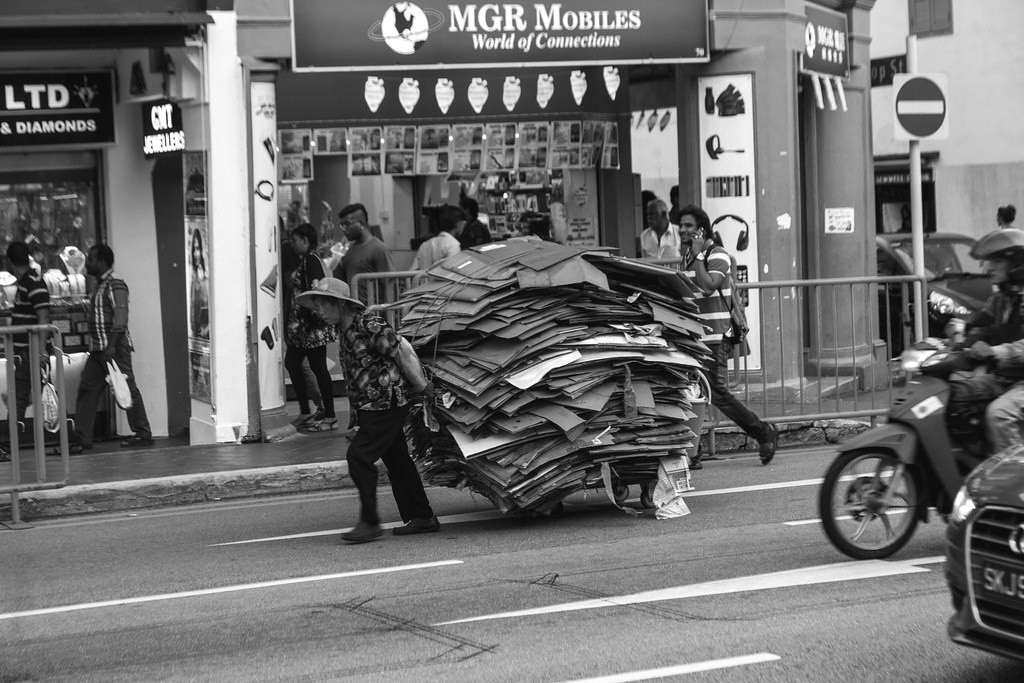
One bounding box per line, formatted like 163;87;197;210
817;313;1020;560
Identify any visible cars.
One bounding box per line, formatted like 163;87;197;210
943;440;1024;663
876;232;998;362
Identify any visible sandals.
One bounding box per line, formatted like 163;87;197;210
291;414;316;430
758;423;778;465
307;419;340;433
687;458;703;470
45;445;81;455
0;449;11;462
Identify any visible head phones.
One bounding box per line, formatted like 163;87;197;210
710;215;749;251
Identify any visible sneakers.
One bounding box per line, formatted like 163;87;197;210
340;519;382;541
393;514;441;534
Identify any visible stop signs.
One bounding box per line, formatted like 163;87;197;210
897;77;948;138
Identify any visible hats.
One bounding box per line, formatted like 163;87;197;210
295;277;365;312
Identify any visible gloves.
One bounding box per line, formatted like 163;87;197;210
422;382;435;399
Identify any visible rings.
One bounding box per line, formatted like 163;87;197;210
695;236;698;238
701;232;703;236
699;235;702;238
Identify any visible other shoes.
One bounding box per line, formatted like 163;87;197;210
307;407;327;421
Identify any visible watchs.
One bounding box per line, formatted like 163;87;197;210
694;252;705;260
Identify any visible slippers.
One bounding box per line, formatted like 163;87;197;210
80;443;92;450
121;433;155;447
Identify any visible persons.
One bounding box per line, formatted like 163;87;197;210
276;216;338;432
457;201;491;249
334;204;398;429
677;205;777;469
190;229;210;398
950;228;1024;451
406;204;460;289
297;277;440;540
636;184;681;270
0;240;60;461
47;243;155;455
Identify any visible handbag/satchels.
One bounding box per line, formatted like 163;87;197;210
40;362;60;433
106;358;133;409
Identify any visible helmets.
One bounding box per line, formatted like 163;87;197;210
970;229;1024;285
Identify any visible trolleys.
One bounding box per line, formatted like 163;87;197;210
424;391;665;516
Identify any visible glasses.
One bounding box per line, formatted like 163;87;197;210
340;220;363;228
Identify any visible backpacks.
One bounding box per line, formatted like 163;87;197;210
703;243;750;344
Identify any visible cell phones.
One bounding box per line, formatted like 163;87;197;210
303;123;619;178
695;227;707;238
736;265;749;306
